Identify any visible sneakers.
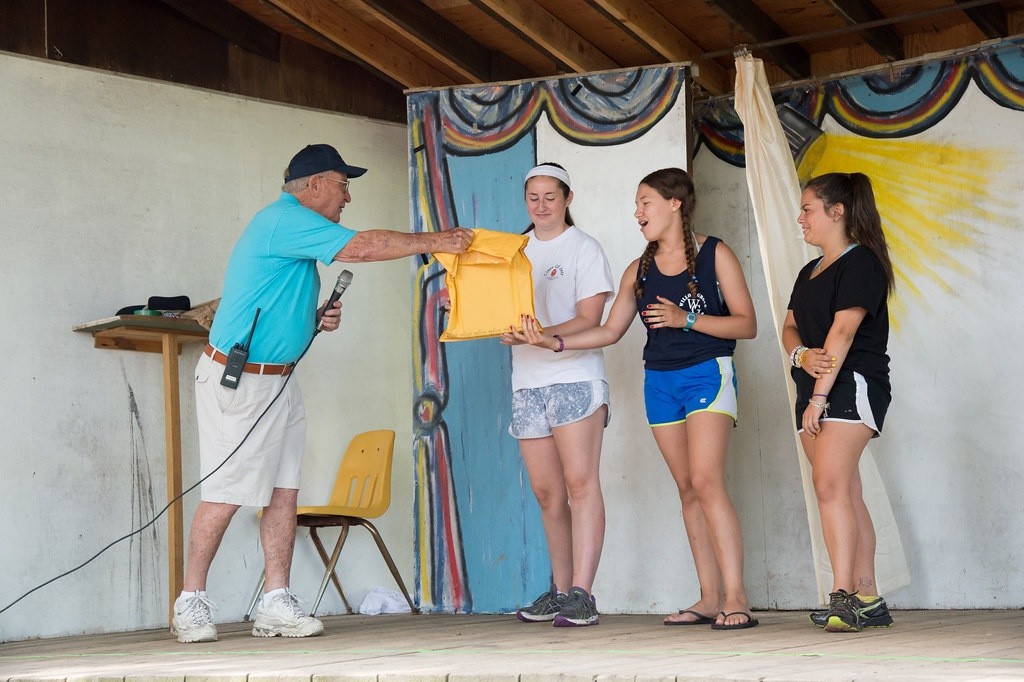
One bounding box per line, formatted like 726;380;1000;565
517;584;568;621
553;587;599;627
252;591;323;637
824;589;863;632
811;597;894;629
170;596;221;643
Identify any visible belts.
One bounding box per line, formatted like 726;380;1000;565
204;343;295;376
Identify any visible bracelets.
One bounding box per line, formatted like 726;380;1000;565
553;334;564;352
809;399;830;417
812;394;828;398
790;346;807;368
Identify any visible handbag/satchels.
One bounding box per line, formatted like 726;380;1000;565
434;229;543;344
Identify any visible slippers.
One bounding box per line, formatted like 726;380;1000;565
665;610;718;627
711;612;759;630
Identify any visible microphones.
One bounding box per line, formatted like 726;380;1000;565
314;270;354;335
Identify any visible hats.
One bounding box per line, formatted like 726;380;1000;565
284;144;368;182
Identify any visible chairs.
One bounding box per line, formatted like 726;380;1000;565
242;429;420;624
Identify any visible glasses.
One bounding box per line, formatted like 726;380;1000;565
307;176;351;193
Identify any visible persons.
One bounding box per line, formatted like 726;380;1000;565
782;171;894;633
171;143;475;642
444;163;615;627
511;168;757;628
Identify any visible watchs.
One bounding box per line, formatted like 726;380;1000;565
684;311;696;332
